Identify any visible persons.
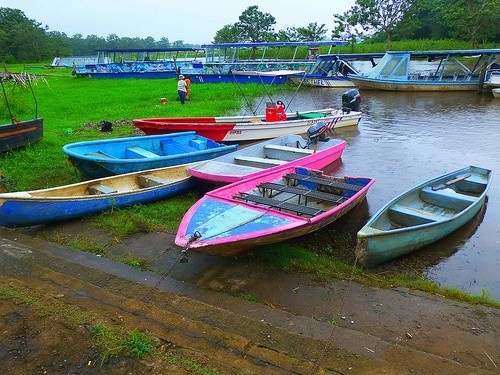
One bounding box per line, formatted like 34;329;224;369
177;75;187;105
184;75;190;101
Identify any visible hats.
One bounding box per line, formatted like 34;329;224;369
179;75;185;79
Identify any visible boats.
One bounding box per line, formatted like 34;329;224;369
0;160;210;229
63;130;240;180
186;122;346;183
403;196;488;270
0;71;45;156
355;164;493;271
132;87;364;145
43;40;386;87
482;69;500;99
346;48;500;91
173;165;375;256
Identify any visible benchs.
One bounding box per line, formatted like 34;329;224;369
235;155;287;168
260;183;344;206
140;174;166;186
421;188;472;210
236;193;321;215
93;151;115;158
390;205;441;226
298;113;326;118
453;174;488;189
90;185;116;194
126;147;159;159
285;174;364;191
264;144;313;159
162;141;196;155
